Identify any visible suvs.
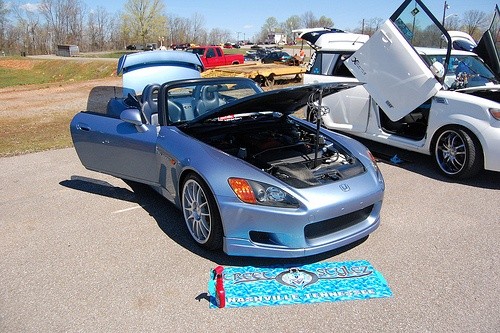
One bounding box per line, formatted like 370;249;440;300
291;0;500;181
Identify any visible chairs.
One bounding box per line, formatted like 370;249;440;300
192;85;226;117
142;83;181;124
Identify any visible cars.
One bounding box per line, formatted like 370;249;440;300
260;51;298;67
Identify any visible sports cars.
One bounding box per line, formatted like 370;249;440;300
71;50;385;259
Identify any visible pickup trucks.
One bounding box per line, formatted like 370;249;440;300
187;45;245;71
